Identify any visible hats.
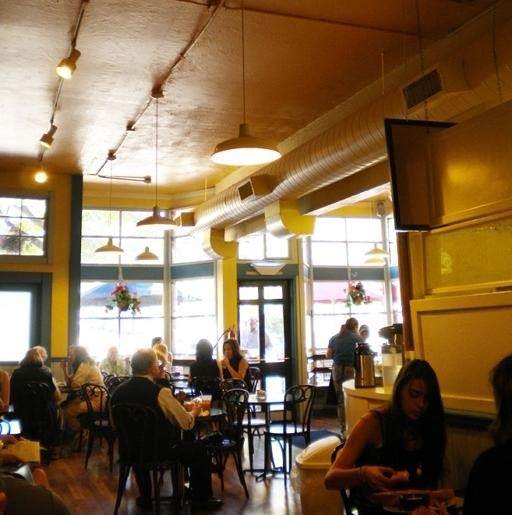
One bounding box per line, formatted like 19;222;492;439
359;325;369;334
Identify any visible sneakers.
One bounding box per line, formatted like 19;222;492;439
51;446;71;459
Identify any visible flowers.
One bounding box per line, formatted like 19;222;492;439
112;284;140;312
348;282;371;306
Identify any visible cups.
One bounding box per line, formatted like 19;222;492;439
382;343;402;394
198;396;212;416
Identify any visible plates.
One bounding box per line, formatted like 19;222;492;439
382;502;450;514
183;374;190;377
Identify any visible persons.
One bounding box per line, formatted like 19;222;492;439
1;336;252;514
460;351;511;514
358;324;369;340
323;357;448;515
242;313;272;349
325;317;365;434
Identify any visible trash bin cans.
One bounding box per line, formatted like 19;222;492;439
295;435;344;515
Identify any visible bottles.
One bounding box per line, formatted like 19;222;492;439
256;390;265;400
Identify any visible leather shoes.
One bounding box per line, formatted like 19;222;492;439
192;496;223;513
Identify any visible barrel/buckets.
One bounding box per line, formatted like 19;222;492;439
381;343;404;387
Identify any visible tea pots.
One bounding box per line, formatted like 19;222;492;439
353;342;375;389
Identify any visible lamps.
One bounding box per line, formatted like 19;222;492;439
211;0;282;166
366;258;386;266
136;99;178;231
39;125;57;149
135;247;159;261
95;159;124;255
366;242;389;257
56;49;80;80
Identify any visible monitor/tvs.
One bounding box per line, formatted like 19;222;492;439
383;116;460;231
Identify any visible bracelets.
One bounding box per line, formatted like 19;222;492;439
359;466;362;476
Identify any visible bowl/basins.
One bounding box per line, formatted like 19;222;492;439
172;373;180;377
400;493;428;510
